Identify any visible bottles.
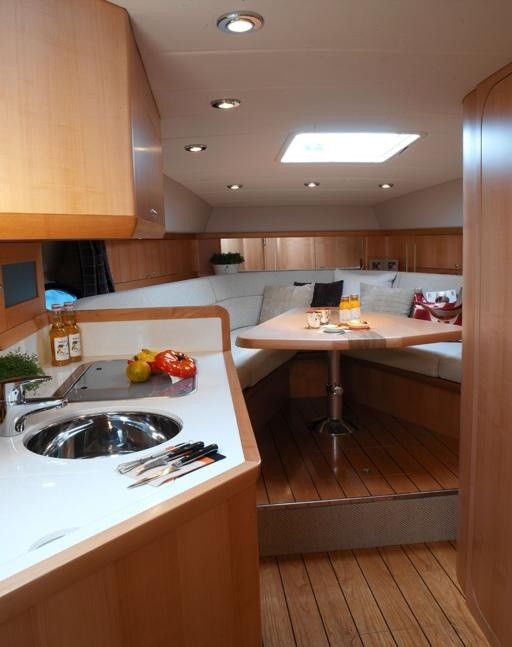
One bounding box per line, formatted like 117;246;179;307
64;302;80;363
413;289;423;305
339;296;349;322
49;304;69;365
350;295;361;322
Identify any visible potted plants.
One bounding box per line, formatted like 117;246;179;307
209;251;244;274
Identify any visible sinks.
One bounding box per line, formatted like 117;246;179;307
22;409;187;460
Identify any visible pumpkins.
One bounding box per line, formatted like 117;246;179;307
154;350;196;379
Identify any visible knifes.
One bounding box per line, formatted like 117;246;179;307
128;444;216;487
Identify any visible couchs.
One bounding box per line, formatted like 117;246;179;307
71;269;463;438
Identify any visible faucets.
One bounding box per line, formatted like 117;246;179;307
0;374;65;436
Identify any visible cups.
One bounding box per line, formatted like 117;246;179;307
307;313;320;328
316;310;330;325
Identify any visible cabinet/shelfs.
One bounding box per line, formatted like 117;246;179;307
0;0;165;240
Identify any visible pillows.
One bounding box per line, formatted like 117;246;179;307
259;269;463;342
